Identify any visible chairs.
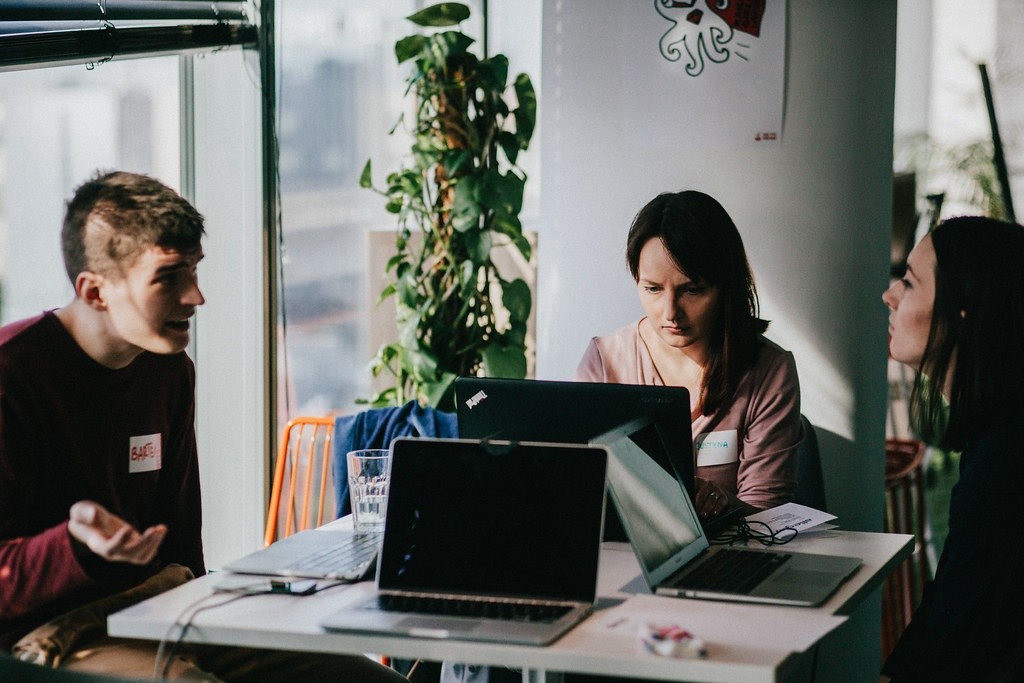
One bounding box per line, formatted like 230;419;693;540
263;417;392;667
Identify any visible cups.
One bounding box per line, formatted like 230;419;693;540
347;449;390;531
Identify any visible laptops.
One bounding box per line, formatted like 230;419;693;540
454;376;742;543
223;528;378;583
588;417;863;607
321;437;610;646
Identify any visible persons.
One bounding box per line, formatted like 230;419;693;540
881;216;1024;683
0;171;412;682
576;191;801;510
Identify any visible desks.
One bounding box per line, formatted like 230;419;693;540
106;513;916;683
